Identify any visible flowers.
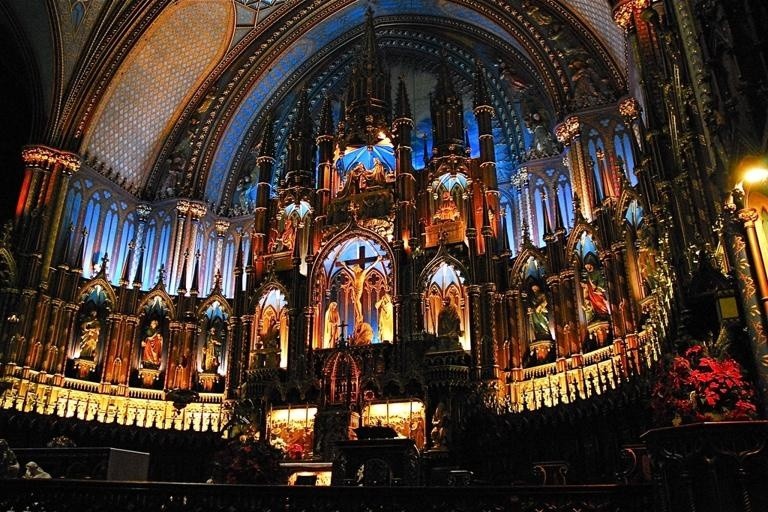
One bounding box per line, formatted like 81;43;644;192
644;327;756;427
